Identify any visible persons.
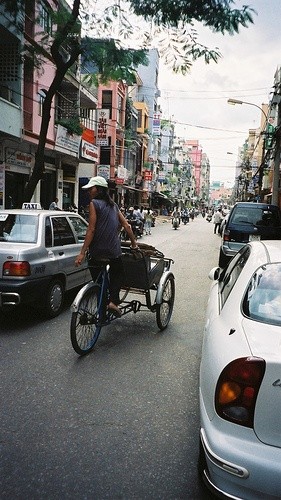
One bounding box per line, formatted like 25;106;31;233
118;204;155;238
212;209;225;234
74;175;140;318
47;197;63;211
170;205;213;235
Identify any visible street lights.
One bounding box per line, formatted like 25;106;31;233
227;97;267;200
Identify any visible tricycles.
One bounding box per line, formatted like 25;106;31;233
67;242;175;354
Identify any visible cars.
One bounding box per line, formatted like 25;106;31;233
195;240;281;496
0;201;93;318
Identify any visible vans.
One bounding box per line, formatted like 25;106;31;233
218;201;280;268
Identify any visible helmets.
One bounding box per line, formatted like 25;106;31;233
129;207;134;210
120;207;125;210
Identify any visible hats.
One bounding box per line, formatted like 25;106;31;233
81;176;108;188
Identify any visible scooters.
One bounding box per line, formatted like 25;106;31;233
119;205;215;242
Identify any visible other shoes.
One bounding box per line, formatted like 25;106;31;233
108;305;121;318
146;234;148;235
149;232;151;235
